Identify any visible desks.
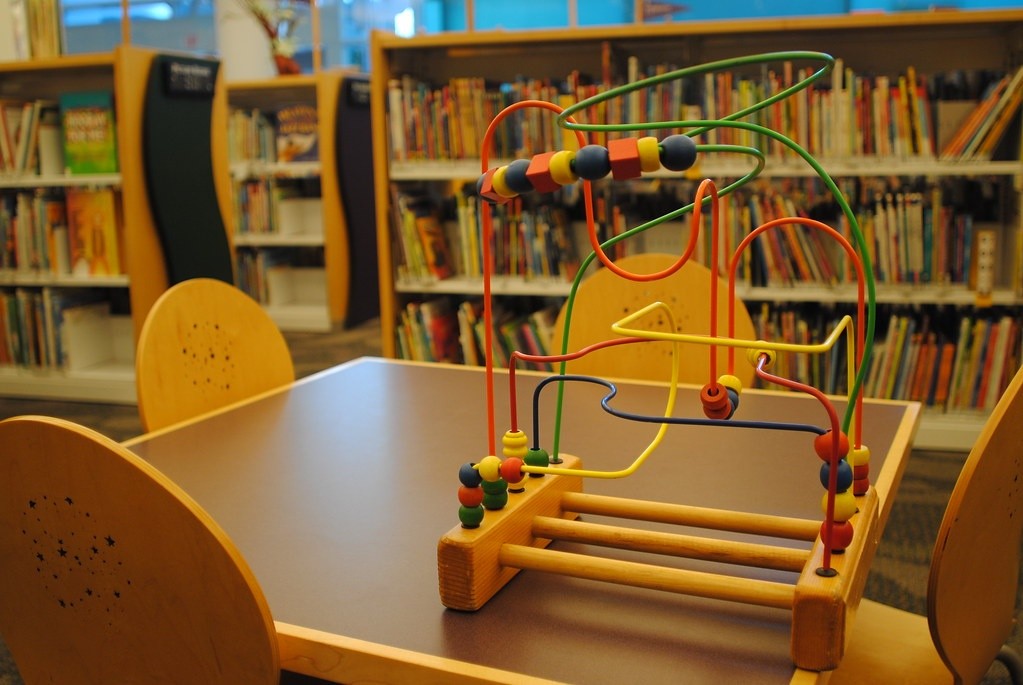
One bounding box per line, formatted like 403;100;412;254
121;358;924;681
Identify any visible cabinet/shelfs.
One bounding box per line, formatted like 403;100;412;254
373;10;1022;450
224;69;368;336
0;48;212;403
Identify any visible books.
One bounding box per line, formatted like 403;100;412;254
0;89;126;367
224;107;301;304
385;59;1023;414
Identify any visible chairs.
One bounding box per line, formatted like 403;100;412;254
2;402;282;685
550;254;762;392
815;370;1023;685
137;278;293;433
